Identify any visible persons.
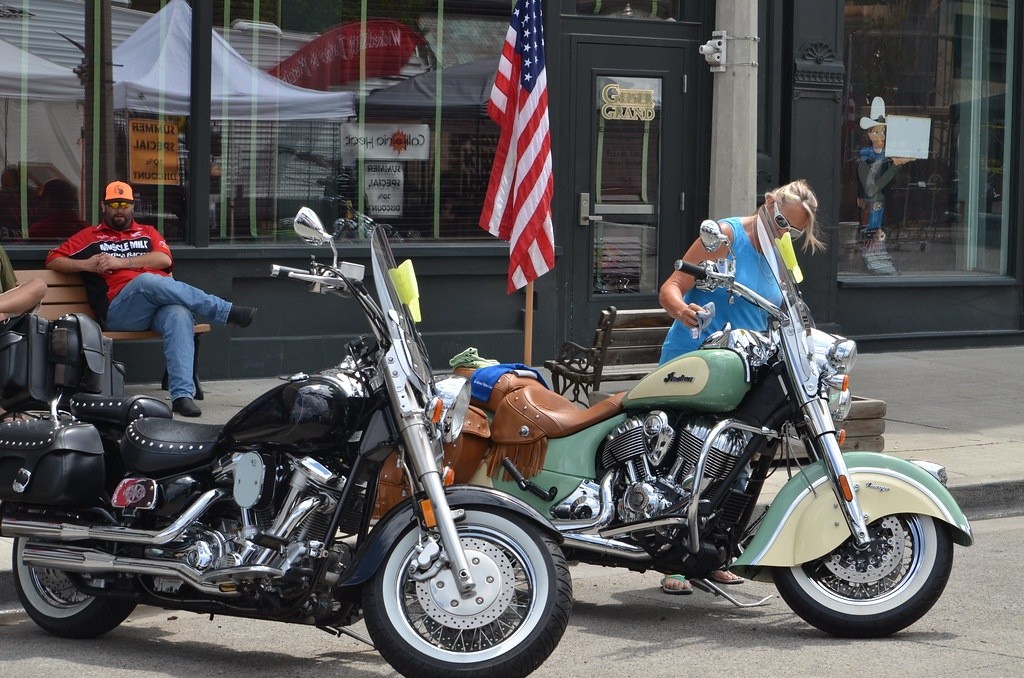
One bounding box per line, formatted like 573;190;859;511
44;179;259;416
24;179;91;240
0;246;47;324
656;176;818;597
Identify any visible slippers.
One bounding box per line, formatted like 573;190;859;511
661;575;693;595
710;564;744;584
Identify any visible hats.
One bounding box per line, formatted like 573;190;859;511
104;181;134;204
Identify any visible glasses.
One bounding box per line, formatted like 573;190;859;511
773;201;805;239
106;201;134;209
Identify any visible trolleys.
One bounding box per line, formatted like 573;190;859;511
876;180;939;253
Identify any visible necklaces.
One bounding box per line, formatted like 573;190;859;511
752;214;775;285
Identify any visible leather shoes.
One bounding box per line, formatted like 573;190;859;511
227;305;258;328
172;397;202;416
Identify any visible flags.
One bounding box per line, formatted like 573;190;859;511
476;1;557;297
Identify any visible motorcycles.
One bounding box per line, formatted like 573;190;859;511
0;205;572;678
371;219;975;637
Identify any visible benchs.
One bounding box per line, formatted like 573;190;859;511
13;270;211;400
543;306;676;409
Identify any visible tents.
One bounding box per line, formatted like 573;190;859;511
357;51;503;115
1;1;357;238
